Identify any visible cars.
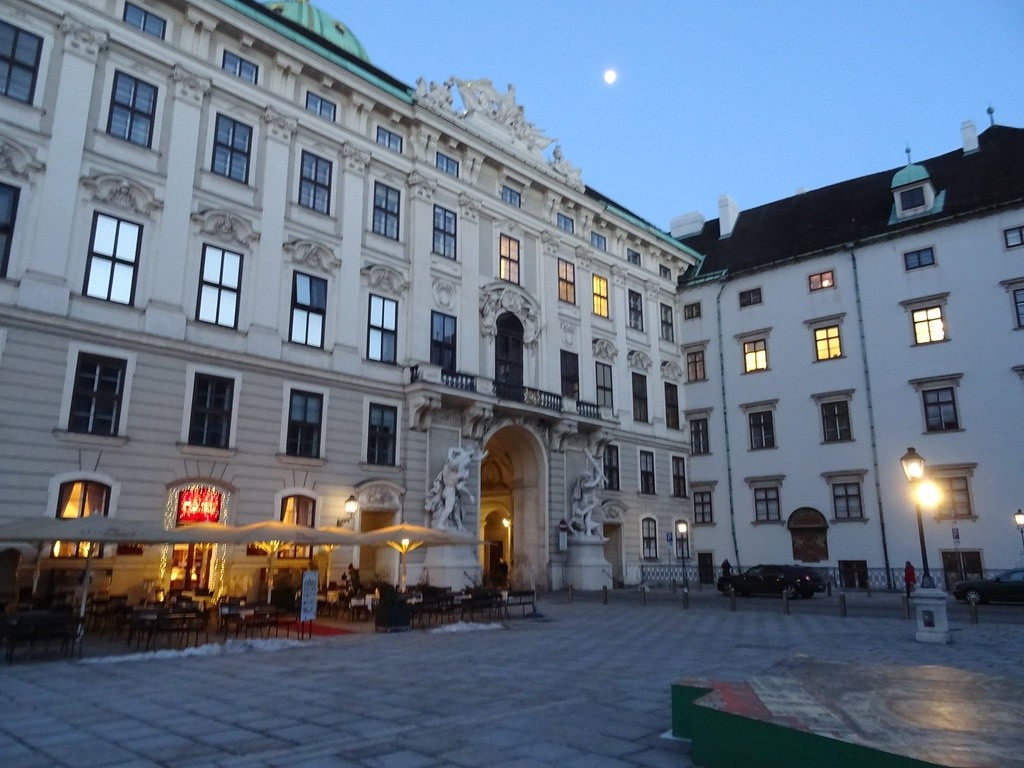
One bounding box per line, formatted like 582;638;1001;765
718;564;826;601
957;570;1023;606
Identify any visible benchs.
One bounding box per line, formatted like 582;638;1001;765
331;590;538;630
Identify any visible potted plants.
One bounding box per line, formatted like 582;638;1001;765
374;584;411;633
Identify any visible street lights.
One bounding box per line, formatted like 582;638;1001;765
898;444;940;591
671;523;689;583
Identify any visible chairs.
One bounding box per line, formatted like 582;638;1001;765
0;593;279;662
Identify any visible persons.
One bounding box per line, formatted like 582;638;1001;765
904;561;917;596
721;558;732;577
436;444;489;533
570;470;610;542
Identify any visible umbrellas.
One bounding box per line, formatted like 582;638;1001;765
0;509;497;624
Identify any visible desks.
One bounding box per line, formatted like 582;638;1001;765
140;612;196;651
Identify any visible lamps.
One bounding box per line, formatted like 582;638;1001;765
503;516;511;526
